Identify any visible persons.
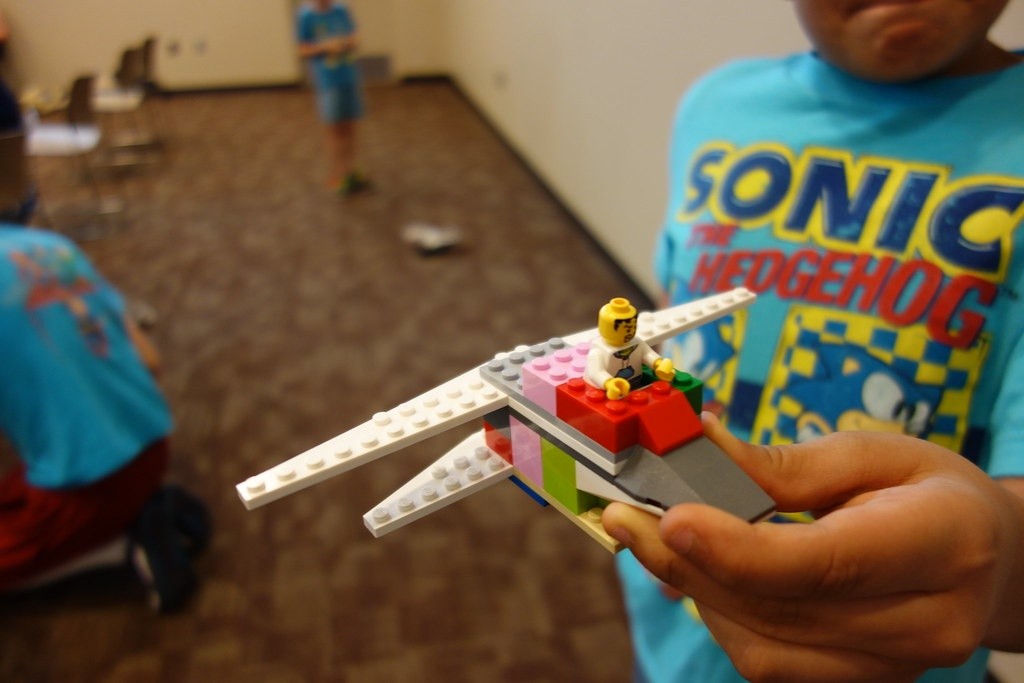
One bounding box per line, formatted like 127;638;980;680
0;228;212;618
602;0;1024;683
0;10;48;227
294;0;376;194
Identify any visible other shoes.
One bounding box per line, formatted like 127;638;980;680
328;158;369;201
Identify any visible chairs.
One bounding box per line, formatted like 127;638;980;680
28;35;166;207
